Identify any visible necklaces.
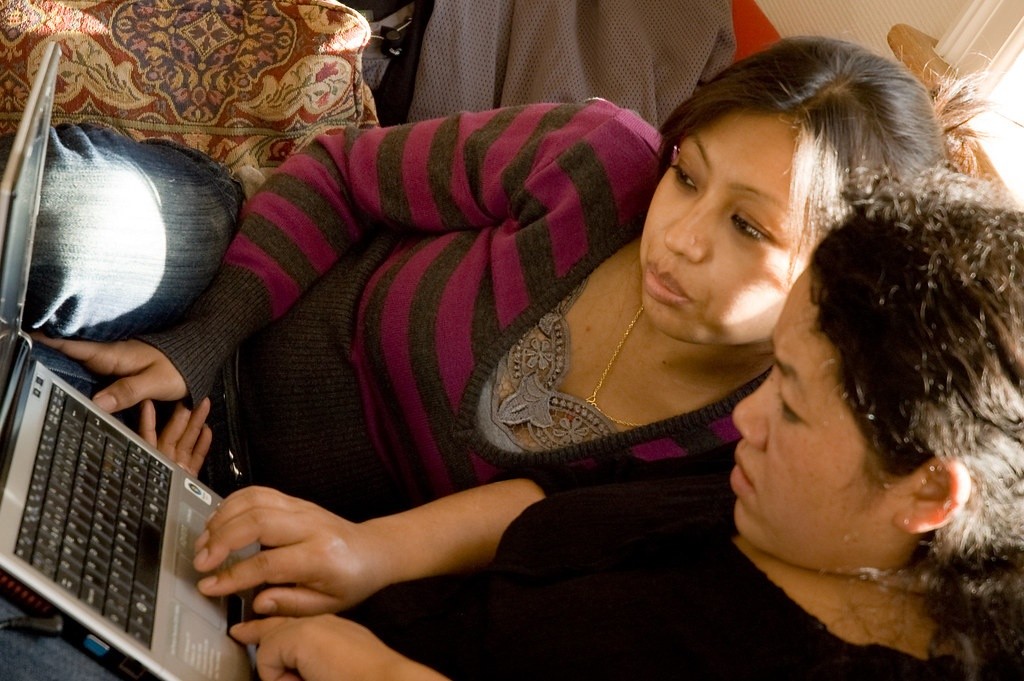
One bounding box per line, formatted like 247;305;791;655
586;305;646;427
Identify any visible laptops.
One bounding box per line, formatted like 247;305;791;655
0;36;262;681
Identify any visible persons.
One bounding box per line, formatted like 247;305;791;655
0;35;957;524
0;177;1024;681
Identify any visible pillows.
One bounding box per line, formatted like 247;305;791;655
0;1;380;179
